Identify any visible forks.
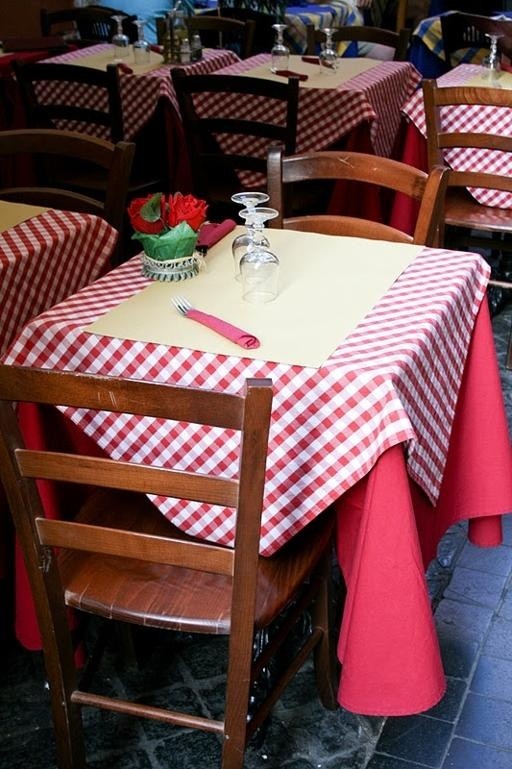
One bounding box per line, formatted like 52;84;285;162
169;293;193;316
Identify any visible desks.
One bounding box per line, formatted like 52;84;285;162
0;200;117;354
8;222;510;712
389;63;512;233
33;41;237;157
190;53;420;216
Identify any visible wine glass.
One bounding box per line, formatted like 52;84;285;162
229;191;280;304
319;28;337;76
270;22;289;75
110;14;149;69
482;30;505;82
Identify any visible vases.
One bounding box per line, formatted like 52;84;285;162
141;253;197;282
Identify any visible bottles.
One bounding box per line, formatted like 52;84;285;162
162;1;202;63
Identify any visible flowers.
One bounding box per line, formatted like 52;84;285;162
126;192;205;257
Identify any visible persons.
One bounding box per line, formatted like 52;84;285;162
74;0;196;45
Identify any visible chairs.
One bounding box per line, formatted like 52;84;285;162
12;58;166;195
1;128;133;234
268;149;448;253
1;1;510;76
2;366;339;767
420;75;510;293
168;67;333;217
